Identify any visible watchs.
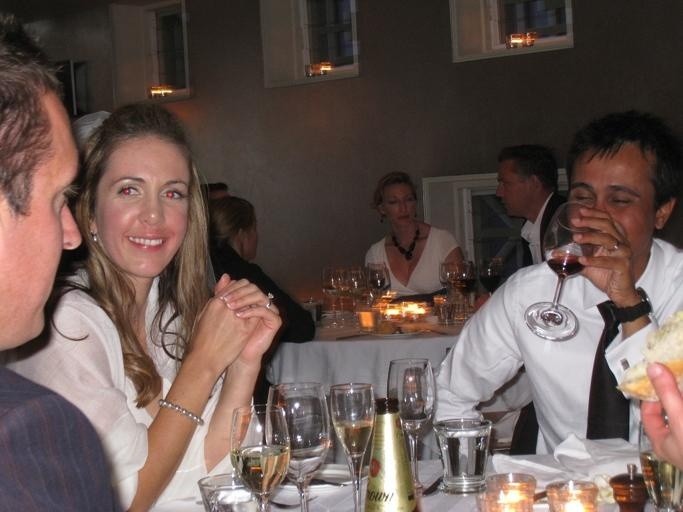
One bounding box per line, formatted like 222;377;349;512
609;286;651;321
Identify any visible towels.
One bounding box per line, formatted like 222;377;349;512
555;433;642;478
492;454;593;494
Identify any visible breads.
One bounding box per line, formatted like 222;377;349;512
614;309;682;401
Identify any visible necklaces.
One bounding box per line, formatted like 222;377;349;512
391;229;420;260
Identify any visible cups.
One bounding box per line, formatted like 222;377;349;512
383;301;432;322
544;480;599;512
476;473;537;512
431;417;494;496
357;307;381;335
196;472;259;512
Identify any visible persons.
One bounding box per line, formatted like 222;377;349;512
640;363;683;471
0;13;122;512
494;144;568;269
364;171;464;300
207;195;315;412
197;183;227;212
424;113;683;455
7;107;283;512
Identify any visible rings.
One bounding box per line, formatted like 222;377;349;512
266;292;274;308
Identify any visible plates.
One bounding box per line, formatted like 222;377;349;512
362;330;426;339
276;462;371;492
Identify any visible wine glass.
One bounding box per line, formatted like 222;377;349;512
385;357;438;498
263;380;332;512
368;262;392;299
637;418;682;511
523;198;602;343
228;404;292;512
321;265;341;329
332;267;352;329
328;381;377;512
438;257;507;318
348;267;367;328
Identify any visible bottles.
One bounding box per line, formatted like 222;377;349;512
364;395;419;512
610;464;647;512
299;295;324;325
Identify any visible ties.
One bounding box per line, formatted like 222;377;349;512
586;301;630;440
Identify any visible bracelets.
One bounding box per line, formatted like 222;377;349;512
157;398;204;425
